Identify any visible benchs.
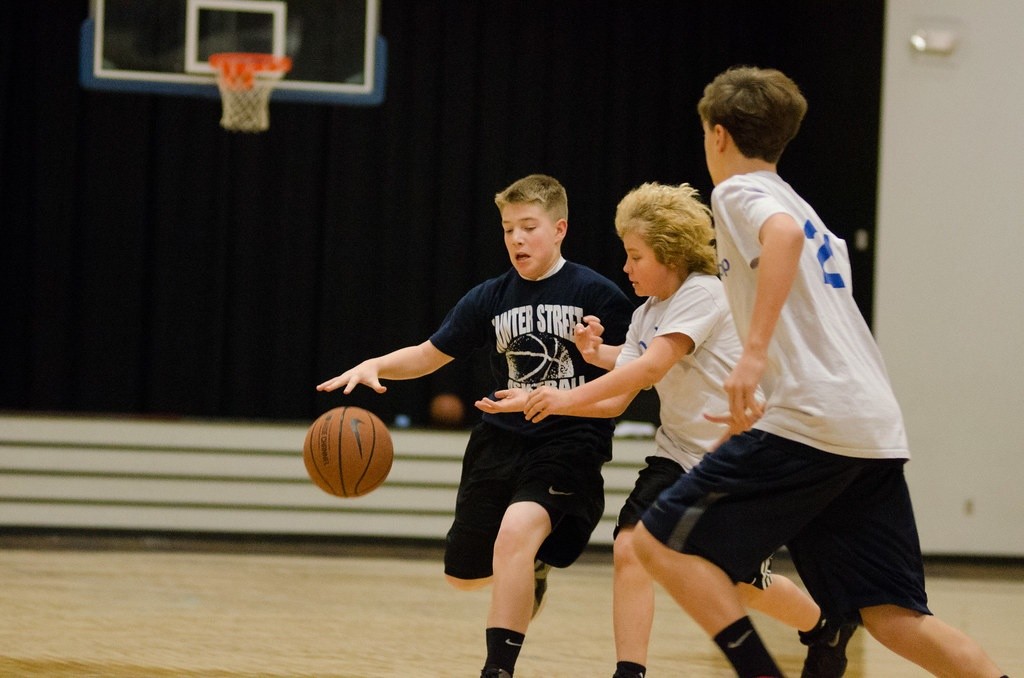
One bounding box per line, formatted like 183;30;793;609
0;414;655;545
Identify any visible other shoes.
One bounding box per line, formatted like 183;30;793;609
480;668;512;678
530;560;551;620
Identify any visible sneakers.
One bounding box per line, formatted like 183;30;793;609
801;619;857;678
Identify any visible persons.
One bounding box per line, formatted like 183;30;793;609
628;67;1012;678
318;172;634;678
472;182;860;678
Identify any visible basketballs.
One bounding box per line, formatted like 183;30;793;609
303;406;394;498
